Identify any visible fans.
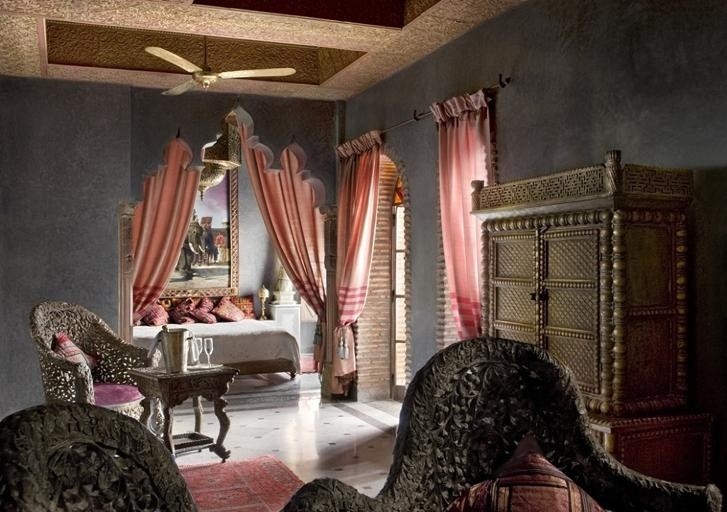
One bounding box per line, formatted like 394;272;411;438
142;35;297;96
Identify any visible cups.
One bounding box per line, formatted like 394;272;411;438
191;337;214;368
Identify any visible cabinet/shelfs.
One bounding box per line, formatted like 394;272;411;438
587;412;713;487
469;148;698;416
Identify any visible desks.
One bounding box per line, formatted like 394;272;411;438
130;366;240;467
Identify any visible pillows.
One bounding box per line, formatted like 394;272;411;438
143;305;169;326
170;296;198;326
211;296;245;322
191;297;218;324
55;330;98;370
447;429;603;512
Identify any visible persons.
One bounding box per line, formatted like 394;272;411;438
177;208;199;280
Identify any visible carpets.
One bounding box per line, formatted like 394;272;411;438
299;354;321;373
178;455;311;512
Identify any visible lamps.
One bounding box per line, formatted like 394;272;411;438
256;284;269;320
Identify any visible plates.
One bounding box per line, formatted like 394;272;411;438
187;364;223;369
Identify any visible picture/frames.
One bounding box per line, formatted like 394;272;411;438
158;168;241;299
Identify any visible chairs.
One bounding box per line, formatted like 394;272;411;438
32;299;160;427
0;403;199;512
280;336;723;512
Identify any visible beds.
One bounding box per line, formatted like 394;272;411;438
133;294;302;378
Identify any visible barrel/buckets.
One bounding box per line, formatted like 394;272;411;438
146;328;190;372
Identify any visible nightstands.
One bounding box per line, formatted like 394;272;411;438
268;303;300;339
